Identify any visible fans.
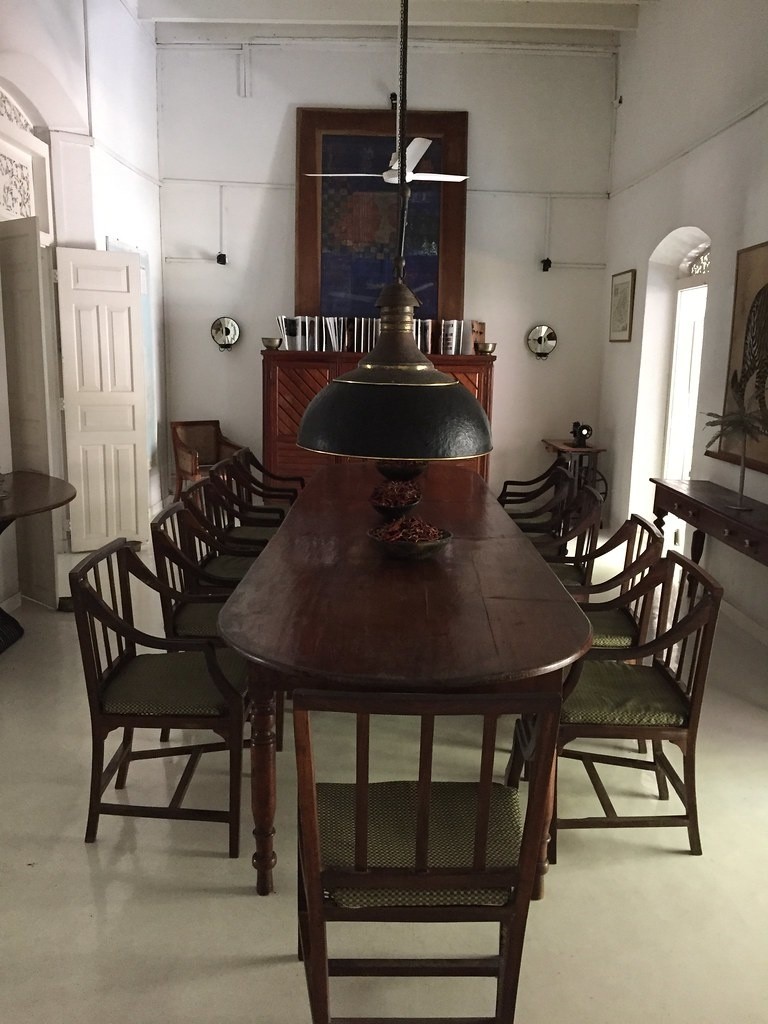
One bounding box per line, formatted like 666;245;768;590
305;93;471;182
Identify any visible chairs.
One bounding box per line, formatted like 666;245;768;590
495;452;723;866
65;441;305;859
168;419;247;503
293;680;564;1024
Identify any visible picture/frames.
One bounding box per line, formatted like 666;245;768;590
716;240;768;475
609;268;637;342
294;106;469;337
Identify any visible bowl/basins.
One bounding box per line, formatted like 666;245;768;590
377;465;424;481
367;527;453;558
373;497;421;516
475;343;496;352
262;338;282;348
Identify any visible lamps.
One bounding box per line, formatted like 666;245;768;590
291;0;500;466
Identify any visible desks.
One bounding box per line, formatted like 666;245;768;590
0;469;79;654
214;462;594;902
645;474;768;597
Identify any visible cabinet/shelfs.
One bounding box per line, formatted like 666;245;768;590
258;347;498;504
